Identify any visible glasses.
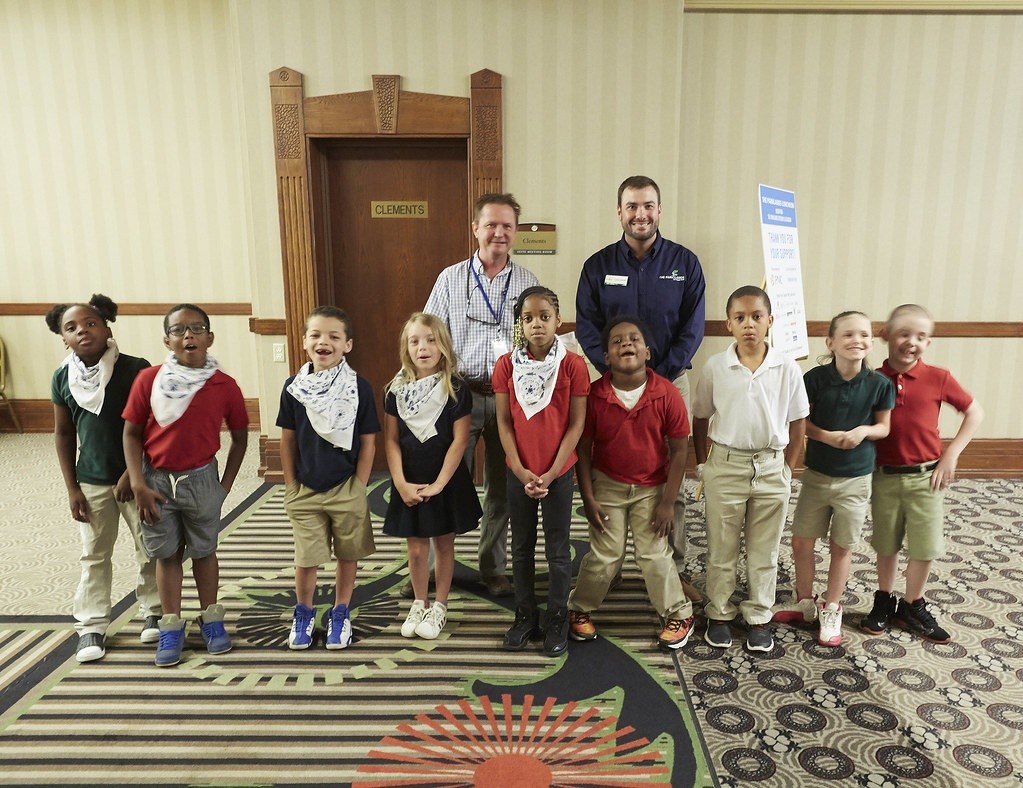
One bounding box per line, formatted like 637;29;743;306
465;284;507;326
167;323;209;336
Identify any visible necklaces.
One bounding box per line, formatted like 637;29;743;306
464;256;512;324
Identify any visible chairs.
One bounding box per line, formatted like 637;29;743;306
0;339;23;434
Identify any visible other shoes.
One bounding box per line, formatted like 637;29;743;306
608;573;623;591
678;570;703;604
478;571;511;595
400;575;436;596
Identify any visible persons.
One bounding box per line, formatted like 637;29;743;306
569;175;707;605
858;304;983;645
44;293;162;664
275;304;383;651
770;310;895;648
690;285;810;653
400;190;544;600
379;311;485;640
566;314;695;651
490;285;591;658
120;304;249;667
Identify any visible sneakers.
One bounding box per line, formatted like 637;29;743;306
400;600;431;638
196;603;233;654
746;621;775;651
658;613;696;649
568;608;597;640
770;593;819;623
864;590;897;634
704;617;733;647
154;614;188;667
326;603;352;650
503;610;541;648
415;600;448;640
140;615;161;643
815;601;843;647
894;598;952;643
287;603;318;650
75;632;108;663
543;617;568;657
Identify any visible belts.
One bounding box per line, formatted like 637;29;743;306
883;460;939;474
469;381;494;396
